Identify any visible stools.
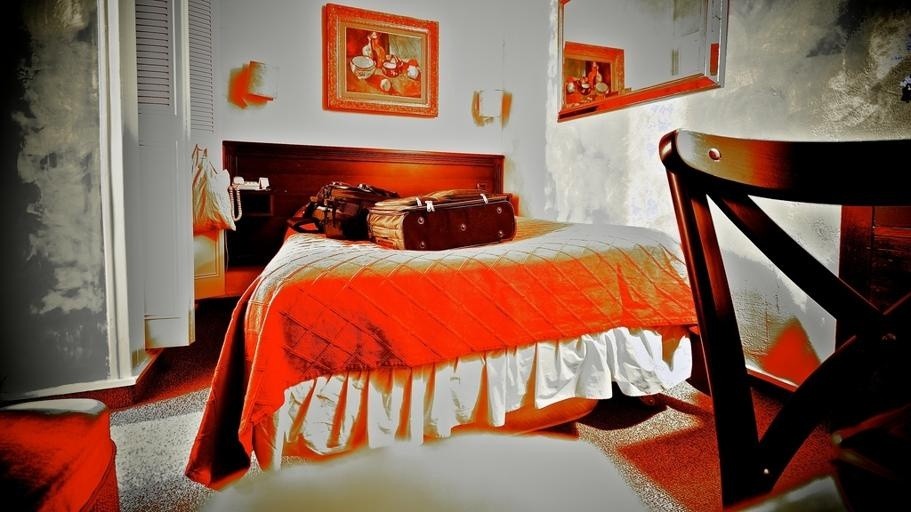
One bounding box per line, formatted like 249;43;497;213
0;405;122;512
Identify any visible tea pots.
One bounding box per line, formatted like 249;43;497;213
361;35;386;68
587;66;602;90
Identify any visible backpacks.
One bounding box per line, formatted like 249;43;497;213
286;181;400;239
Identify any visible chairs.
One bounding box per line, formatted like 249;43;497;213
659;129;911;512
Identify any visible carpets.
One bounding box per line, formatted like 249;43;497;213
109;384;835;512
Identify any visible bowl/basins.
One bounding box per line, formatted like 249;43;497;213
350;54;377;80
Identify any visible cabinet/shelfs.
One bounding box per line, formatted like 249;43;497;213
670;0;712;81
1;0;223;412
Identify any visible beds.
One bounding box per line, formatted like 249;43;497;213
219;138;702;486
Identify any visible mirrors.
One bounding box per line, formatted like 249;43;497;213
555;0;727;123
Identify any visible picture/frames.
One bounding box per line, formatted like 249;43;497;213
561;40;626;111
324;3;440;119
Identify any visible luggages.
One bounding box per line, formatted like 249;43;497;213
367;189;518;251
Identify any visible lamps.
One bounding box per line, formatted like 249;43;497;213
475;89;507;123
241;60;281;103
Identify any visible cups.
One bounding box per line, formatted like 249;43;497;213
594;82;609;96
578;83;591;95
381;60;399;78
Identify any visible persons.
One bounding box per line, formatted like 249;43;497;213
362;31;402;69
581;60;601;88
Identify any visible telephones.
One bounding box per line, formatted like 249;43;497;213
232;176;259;191
